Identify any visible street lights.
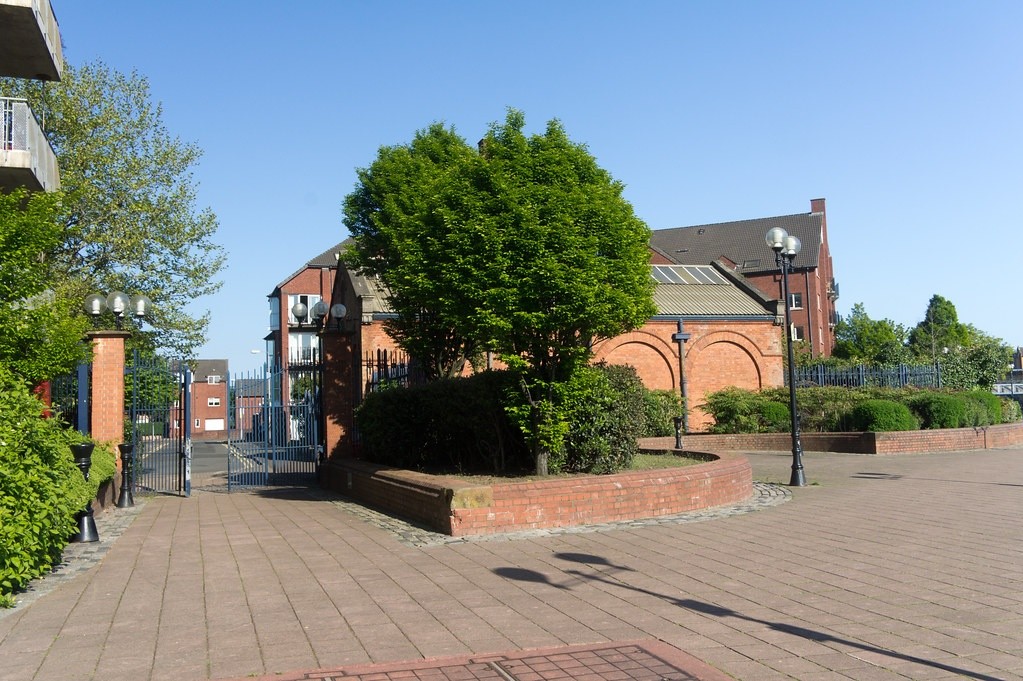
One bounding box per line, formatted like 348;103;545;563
1006;364;1015;401
766;228;807;489
84;290;151;330
291;301;347;491
